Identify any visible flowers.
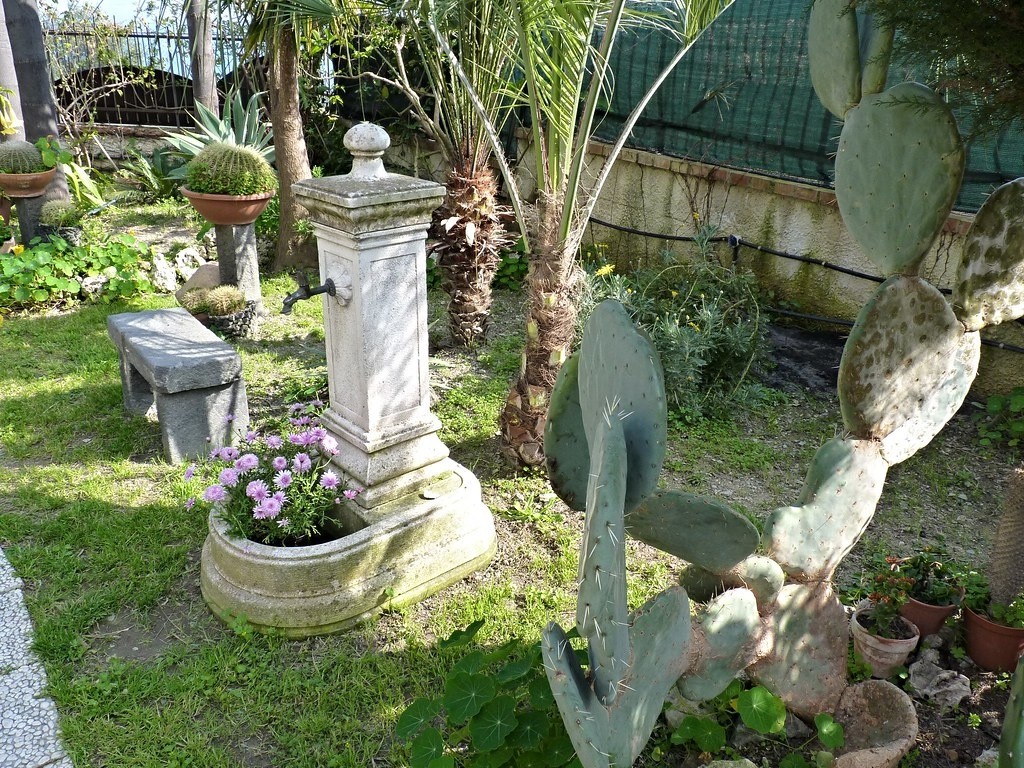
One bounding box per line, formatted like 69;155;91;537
866;551;915;637
907;532;968;605
187;398;368;535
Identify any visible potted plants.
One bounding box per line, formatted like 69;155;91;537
0;139;56;198
183;287;211;328
206;285;256;338
180;142;277;225
960;468;1024;672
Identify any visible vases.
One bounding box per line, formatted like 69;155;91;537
887;556;967;636
852;608;921;680
